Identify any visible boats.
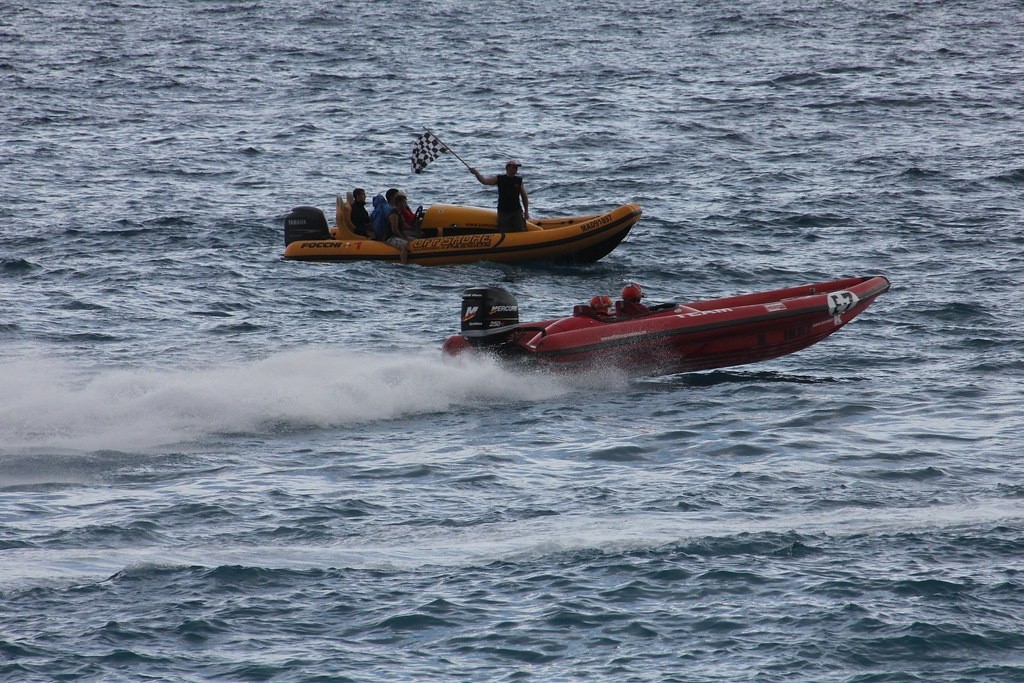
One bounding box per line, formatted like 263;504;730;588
281;201;643;268
441;275;891;381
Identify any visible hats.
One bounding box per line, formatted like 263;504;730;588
507;161;521;167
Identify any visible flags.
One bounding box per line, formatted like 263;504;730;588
410;132;447;173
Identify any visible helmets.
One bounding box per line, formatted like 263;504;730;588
590;296;612;309
622;283;641;298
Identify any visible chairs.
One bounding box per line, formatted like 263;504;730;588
336;194;369;240
347;192;355;208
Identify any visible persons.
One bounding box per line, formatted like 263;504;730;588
619;283;652;316
367;194;410;265
392;195;436;239
469;160;530;232
384;188;412;231
349;188;373;234
589;296;613;320
